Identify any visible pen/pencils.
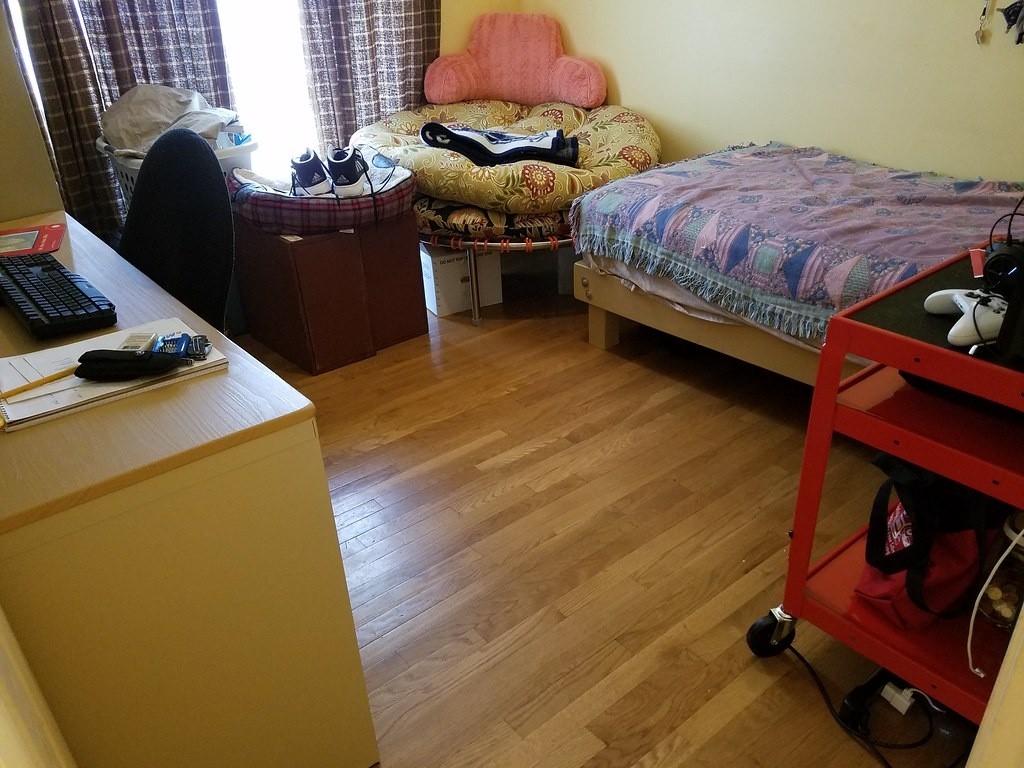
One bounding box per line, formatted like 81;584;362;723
0;363;80;402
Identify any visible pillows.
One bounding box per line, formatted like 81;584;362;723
424;11;607;109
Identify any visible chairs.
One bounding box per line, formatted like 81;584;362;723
117;128;235;334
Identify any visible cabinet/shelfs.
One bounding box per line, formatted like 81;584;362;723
0;210;380;768
746;235;1024;725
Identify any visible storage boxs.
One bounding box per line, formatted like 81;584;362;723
557;246;582;294
358;211;429;350
235;221;376;375
419;243;504;318
95;135;259;207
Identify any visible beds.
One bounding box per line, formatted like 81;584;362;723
568;139;1024;387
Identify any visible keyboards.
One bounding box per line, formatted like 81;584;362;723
0;252;117;336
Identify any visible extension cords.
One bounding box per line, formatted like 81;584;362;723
838;667;911;729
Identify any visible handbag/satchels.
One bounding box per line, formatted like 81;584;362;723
856;451;1012;632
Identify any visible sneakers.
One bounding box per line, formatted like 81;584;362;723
289;148;333;196
327;143;379;233
